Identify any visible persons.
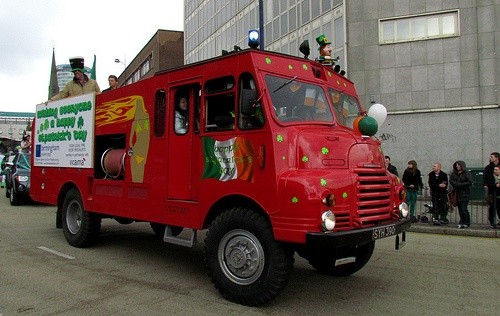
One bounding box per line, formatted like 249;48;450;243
384;155;399;179
101;74;119;92
316;34;345;76
0;135;32;188
44;67;102;106
428;162;449;226
449;160;474;228
174;93;189;135
482;151;500;230
402;160;424;222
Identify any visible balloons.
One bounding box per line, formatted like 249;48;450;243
366;103;388;131
352;115;366;135
358;115;378;137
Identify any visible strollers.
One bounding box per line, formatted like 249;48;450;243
417;188;453;223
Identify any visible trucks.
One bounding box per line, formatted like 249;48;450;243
27;29;412;308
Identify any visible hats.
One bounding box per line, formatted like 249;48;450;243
69;58;87;73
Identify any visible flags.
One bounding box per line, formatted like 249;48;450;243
48;48;60;101
90;54;96;80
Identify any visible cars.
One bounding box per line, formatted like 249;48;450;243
3;152;32;206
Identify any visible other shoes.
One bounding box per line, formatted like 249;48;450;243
438;220;444;225
462;224;468;228
491;225;496;229
433;219;439;225
458;223;462;228
